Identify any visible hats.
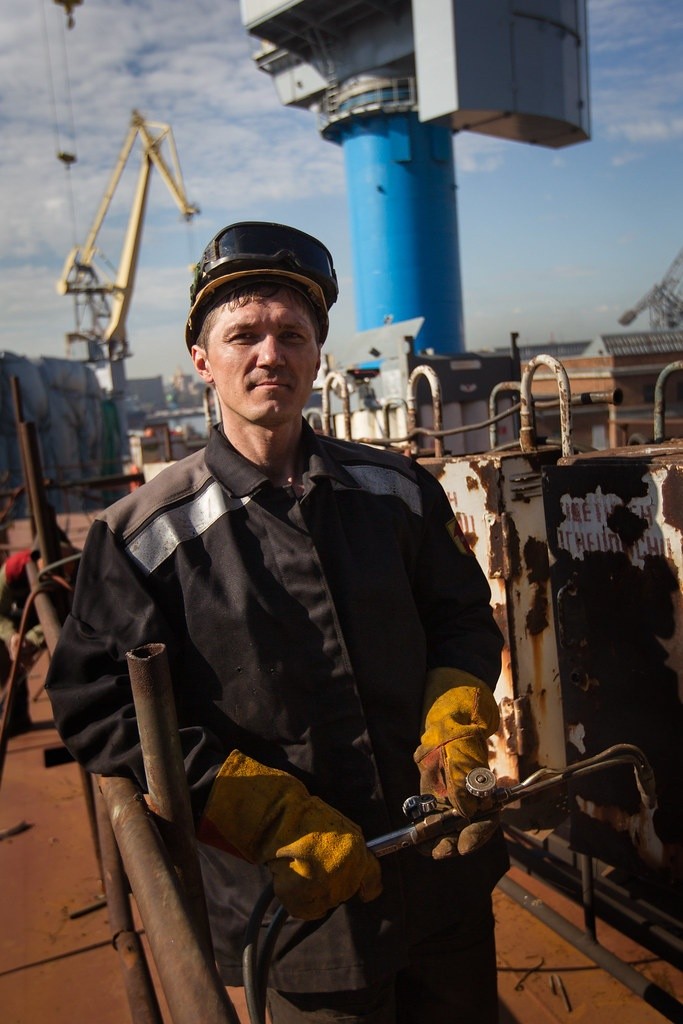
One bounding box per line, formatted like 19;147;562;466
28;524;82;564
186;221;339;355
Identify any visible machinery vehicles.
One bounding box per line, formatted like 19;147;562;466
40;0;203;365
618;245;683;334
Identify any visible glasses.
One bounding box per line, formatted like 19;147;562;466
189;222;337;288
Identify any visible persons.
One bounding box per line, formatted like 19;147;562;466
43;220;504;1024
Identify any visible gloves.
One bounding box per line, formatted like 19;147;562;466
405;666;500;861
200;748;385;920
